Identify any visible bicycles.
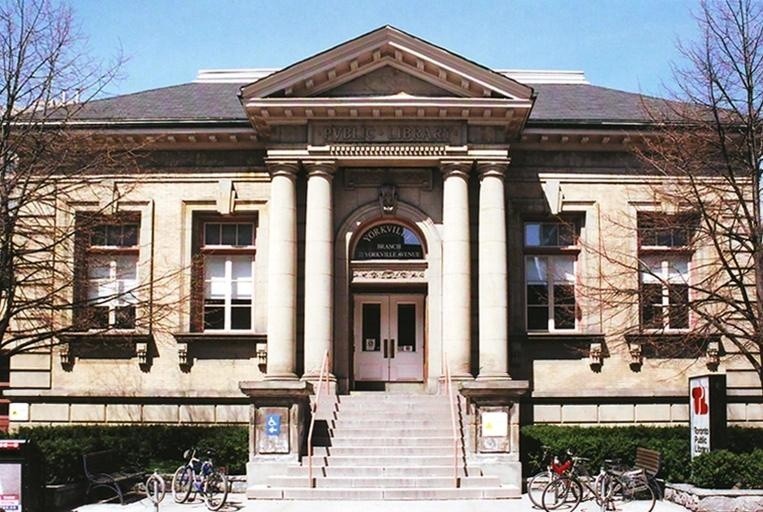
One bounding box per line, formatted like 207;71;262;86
170;443;229;511
528;445;592;509
541;449;656;512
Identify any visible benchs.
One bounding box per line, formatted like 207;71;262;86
83;449;153;506
616;447;663;504
